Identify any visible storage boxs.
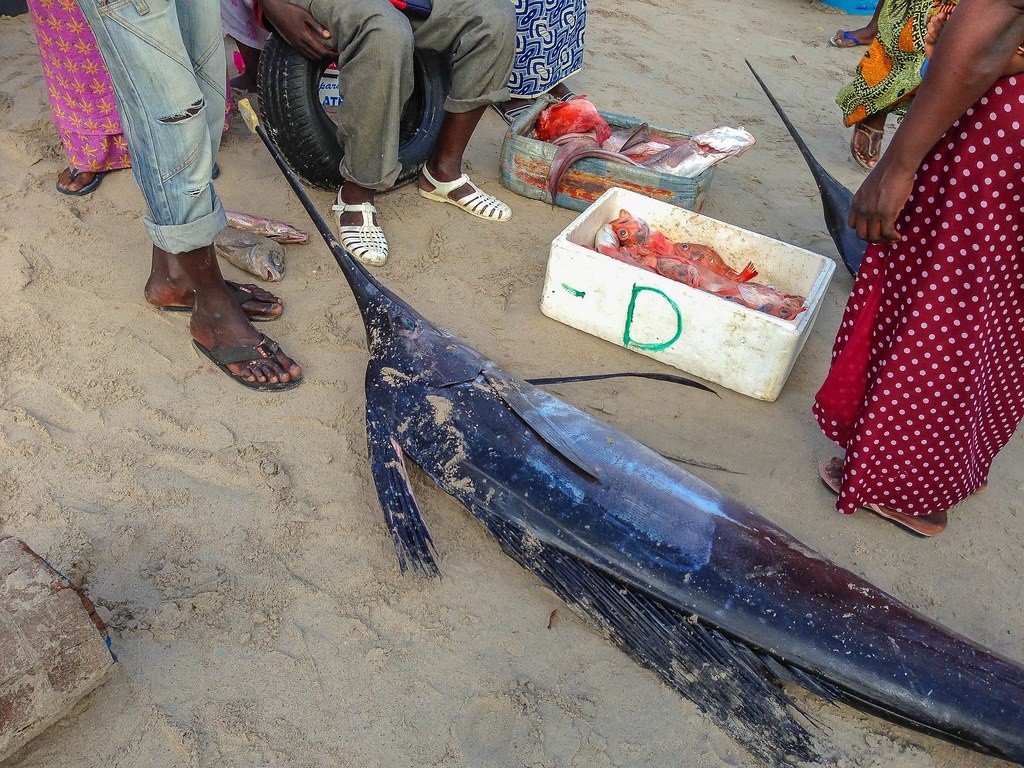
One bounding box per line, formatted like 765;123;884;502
500;96;721;216
543;188;837;404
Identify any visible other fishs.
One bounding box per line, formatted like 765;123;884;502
531;94;756;213
580;209;809;320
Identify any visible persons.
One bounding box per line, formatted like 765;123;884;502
835;0;960;170
246;0;516;266
811;0;1024;538
829;0;884;47
27;0;231;196
73;0;303;392
220;0;271;93
492;0;588;125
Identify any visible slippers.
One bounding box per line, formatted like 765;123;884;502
849;121;884;171
830;30;861;47
212;162;219;179
975;482;989;494
193;332;304;391
156;276;284;322
491;101;532;127
558;90;577;102
56;167;113;196
818;457;936;538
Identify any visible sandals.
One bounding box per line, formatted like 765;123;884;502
417;162;513;222
332;185;389;266
